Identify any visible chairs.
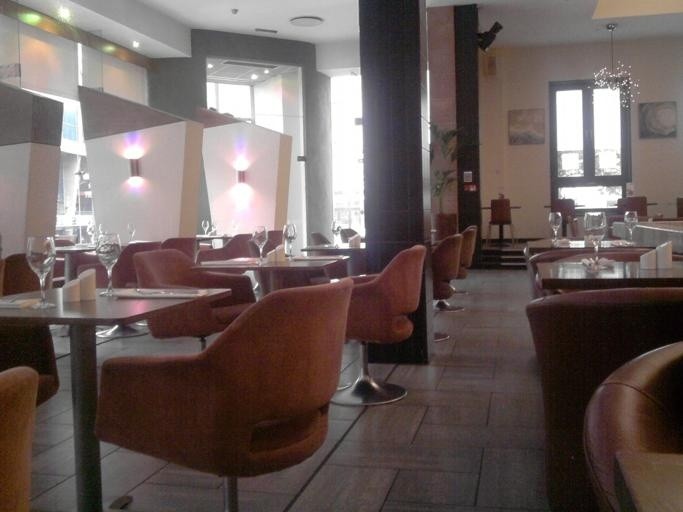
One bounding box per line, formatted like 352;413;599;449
329;243;427;408
522;239;683;512
0;228;366;352
616;197;648;217
488;199;513;247
93;278;354;512
429;212;477;313
550;198;576;237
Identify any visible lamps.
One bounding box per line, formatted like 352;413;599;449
349;144;363;163
124;145;145;187
587;23;640;113
228;155;253;211
290;16;323;27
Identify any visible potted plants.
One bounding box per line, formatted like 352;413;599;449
422;116;483;241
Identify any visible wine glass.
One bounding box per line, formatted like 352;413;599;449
548;212;563;246
200;218;219;236
282;223;297;261
126;223;136;243
582;211;613;271
251;224;269;265
85;223;109;248
330;219;343;248
94;232;125;298
24;235;62;310
624;210;639;246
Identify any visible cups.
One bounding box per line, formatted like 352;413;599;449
212;238;223;249
